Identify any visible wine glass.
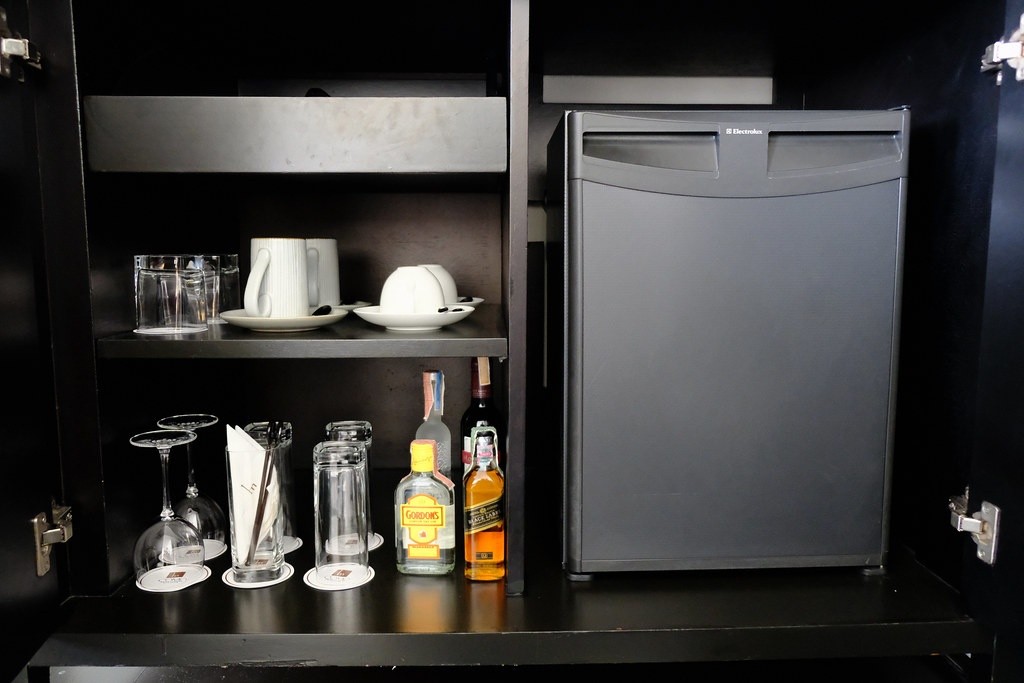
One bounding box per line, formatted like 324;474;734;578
131;429;205;590
154;414;226;563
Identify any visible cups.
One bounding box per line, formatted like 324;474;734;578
242;238;312;317
306;236;339;307
134;254;182;333
226;439;281;582
175;256;219;326
210;253;241;309
380;267;444;315
316;441;369;583
417;264;457;304
244;421;293;552
327;420;373;553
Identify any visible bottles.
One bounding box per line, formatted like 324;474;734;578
414;370;452;477
458;356;504;473
396;440;457;575
461;448;509;582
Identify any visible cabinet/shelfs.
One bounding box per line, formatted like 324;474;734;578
0;0;1024;683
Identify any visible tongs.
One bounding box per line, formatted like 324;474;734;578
244;421;284;564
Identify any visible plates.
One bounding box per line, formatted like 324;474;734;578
457;297;485;306
217;308;345;333
335;301;374;310
351;307;474;333
